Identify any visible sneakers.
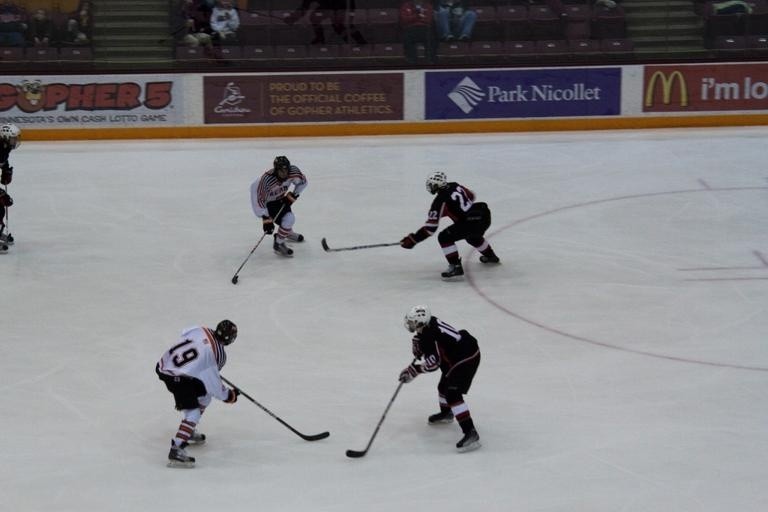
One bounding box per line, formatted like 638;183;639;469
0;233;14;250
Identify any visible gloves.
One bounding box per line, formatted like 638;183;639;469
401;233;419;249
0;190;13;206
281;192;299;207
0;167;13;185
262;215;274;234
223;388;240;404
412;335;423;360
398;363;424;383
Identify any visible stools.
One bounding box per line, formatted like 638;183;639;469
695;0;768;62
167;0;638;74
0;0;93;74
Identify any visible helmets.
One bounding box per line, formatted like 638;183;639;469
0;123;22;149
425;171;447;195
215;320;237;344
274;156;291;172
404;304;431;333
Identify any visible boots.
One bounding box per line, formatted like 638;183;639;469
456;416;479;448
429;406;454;422
168;439;195;463
273;233;293;255
480;250;499;263
288;232;303;241
190;434;206;440
441;258;464;277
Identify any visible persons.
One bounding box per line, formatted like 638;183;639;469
210;0;242;40
0;123;23;252
390;0;441;65
172;0;218;63
250;155;307;256
398;304;480;450
20;8;54;49
153;318;241;465
56;16;87;42
401;172;499;277
432;0;477;57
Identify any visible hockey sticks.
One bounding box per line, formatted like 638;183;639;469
1;183;10;249
321;237;403;251
232;206;284;283
219;374;329;441
346;356;418;456
